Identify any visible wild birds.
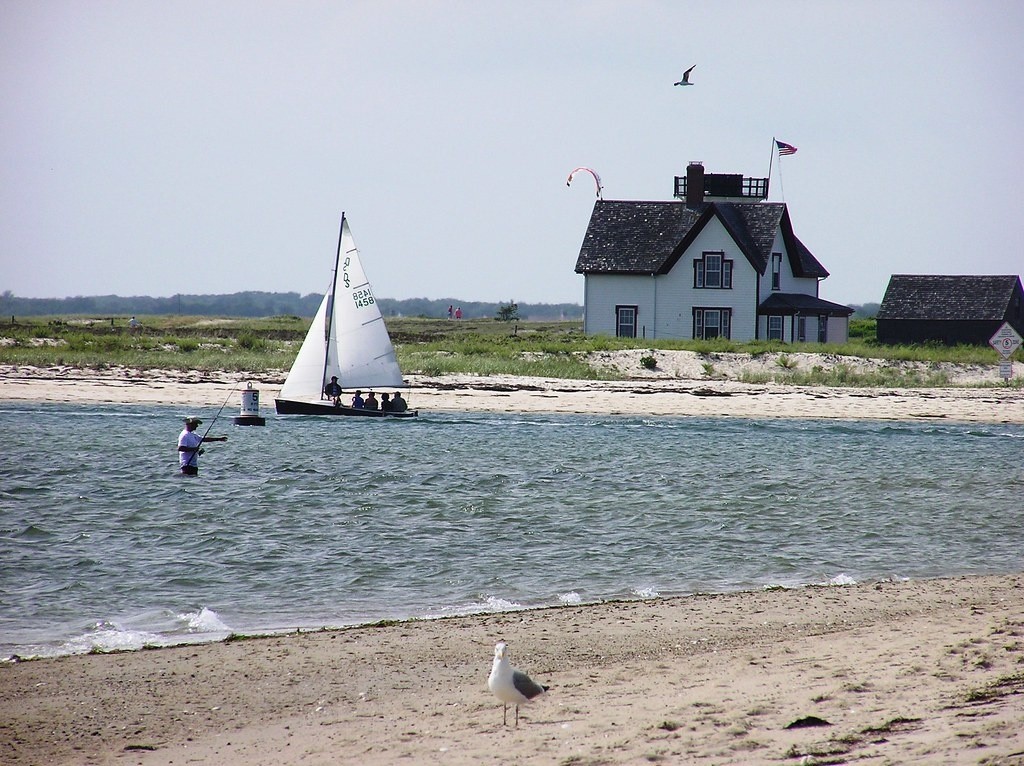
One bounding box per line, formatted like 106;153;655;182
673;64;696;86
487;643;550;726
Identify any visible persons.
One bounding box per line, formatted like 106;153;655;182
129;317;136;327
178;416;228;475
391;392;407;412
381;393;391;410
350;390;364;408
455;307;462;319
325;376;342;396
364;392;378;410
448;305;452;320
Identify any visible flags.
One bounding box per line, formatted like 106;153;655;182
776;140;798;156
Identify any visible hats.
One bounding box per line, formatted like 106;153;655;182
331;376;339;380
186;416;203;425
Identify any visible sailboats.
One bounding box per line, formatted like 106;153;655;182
274;212;420;419
